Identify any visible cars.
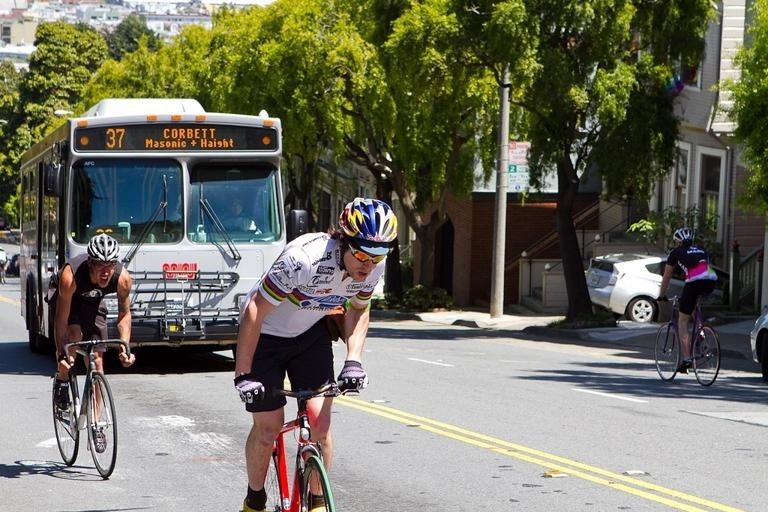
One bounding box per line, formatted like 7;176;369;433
747;302;768;384
585;249;743;324
0;227;21;276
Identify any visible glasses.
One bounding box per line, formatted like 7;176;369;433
346;243;386;264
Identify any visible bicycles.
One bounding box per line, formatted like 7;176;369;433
257;378;344;512
0;268;7;284
51;337;131;481
651;292;723;389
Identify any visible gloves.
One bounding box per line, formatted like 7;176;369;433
657;295;669;304
234;373;264;403
337;360;368;394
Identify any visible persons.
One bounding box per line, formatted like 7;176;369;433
660;225;718;372
210;198;262;234
233;196;400;511
49;233;137;454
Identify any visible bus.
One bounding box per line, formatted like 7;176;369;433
14;94;310;360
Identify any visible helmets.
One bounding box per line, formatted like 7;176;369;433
339;197;398;247
86;234;120;264
673;228;693;243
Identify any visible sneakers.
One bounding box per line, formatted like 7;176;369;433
243;496;267;512
90;424;108;452
676;358;693;372
54;379;71;409
307;502;330;512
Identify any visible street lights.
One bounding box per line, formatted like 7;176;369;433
0;119;10;126
54;110;76;118
